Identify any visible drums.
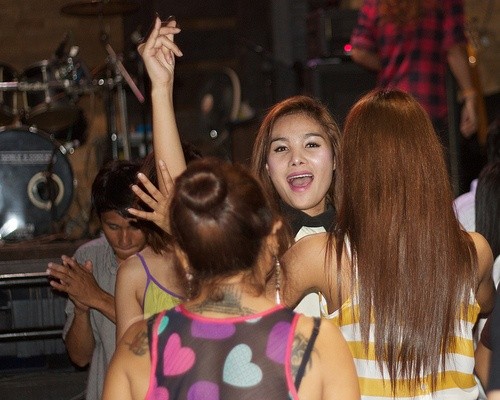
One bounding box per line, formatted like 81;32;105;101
16;60;89;150
0;61;17;125
0;125;78;243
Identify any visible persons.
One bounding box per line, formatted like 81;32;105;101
46;162;148;400
474;282;500;400
453;163;500;260
117;220;195;348
351;1;488;148
102;162;362;400
137;17;342;317
264;90;496;400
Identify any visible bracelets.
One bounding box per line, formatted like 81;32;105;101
460;79;478;94
